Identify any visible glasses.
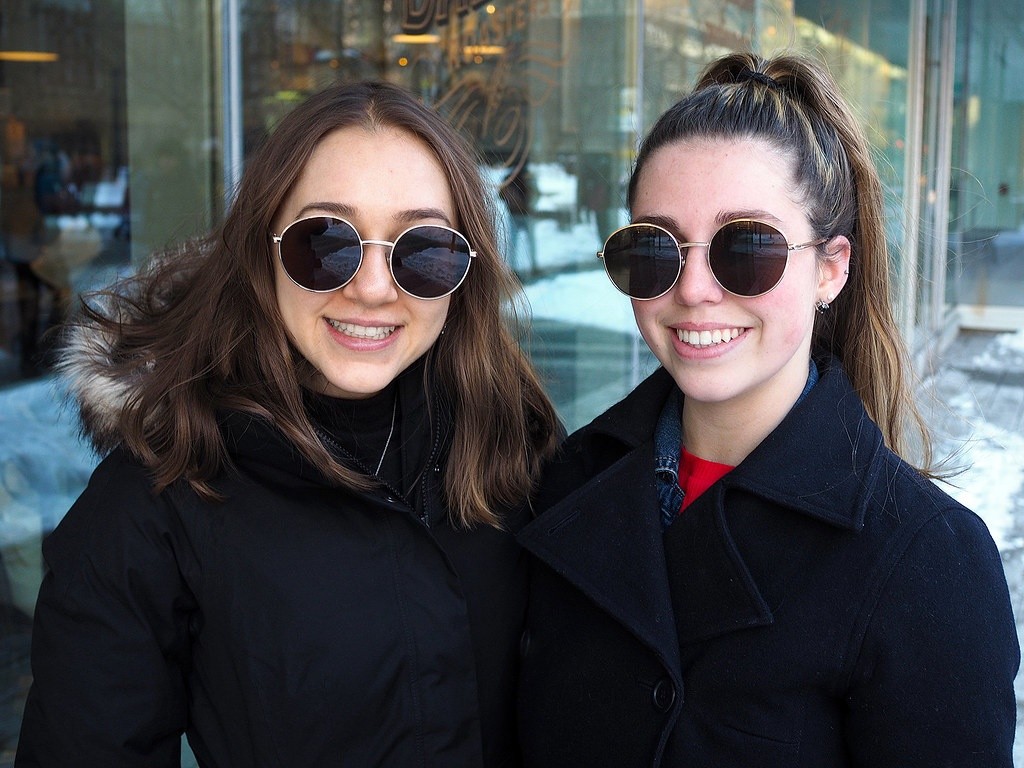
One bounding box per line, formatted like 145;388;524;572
268;215;476;301
596;218;830;300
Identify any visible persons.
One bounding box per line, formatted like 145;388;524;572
15;83;566;768
519;46;1020;768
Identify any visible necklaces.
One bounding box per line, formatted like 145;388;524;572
375;398;396;477
